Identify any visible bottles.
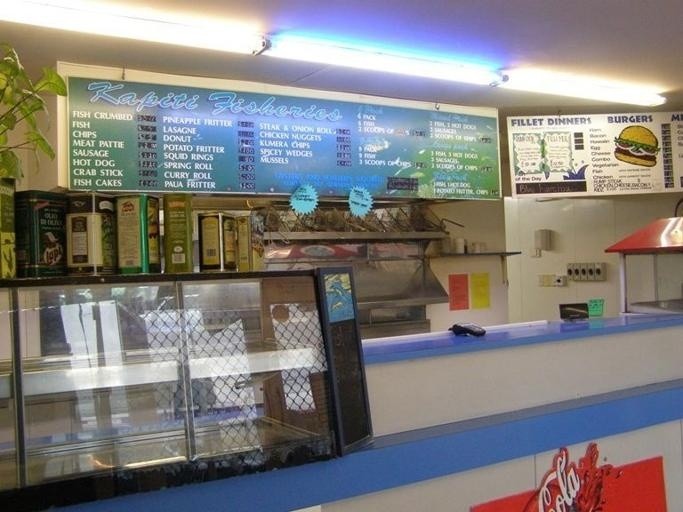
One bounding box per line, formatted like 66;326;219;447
472;242;480;253
453;238;465;253
441;231;451;253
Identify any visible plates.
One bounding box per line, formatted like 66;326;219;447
300;245;336;256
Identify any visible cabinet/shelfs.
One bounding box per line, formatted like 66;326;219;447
256;200;450;339
1;266;373;511
